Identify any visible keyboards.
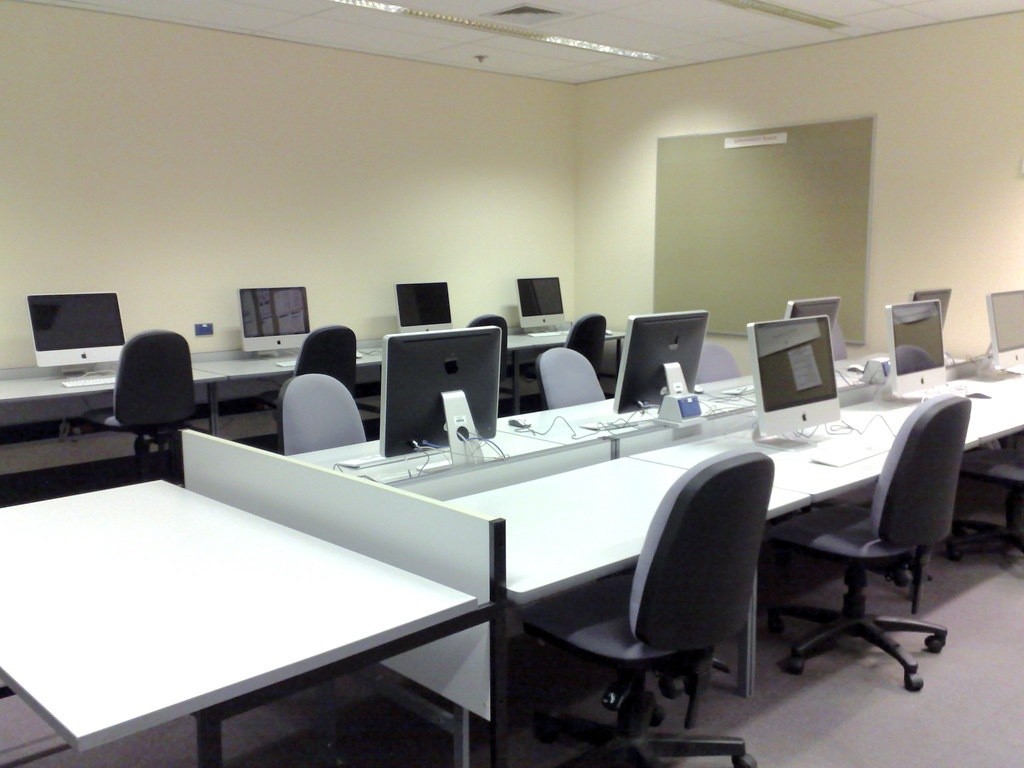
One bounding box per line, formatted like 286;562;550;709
721;385;754;395
276;361;296;367
579;412;657;431
811;429;900;468
62;378;116;388
336;446;448;468
528;331;570;337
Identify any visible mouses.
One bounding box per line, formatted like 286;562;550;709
508;419;531;428
695;385;703;393
605;330;614;335
846;365;865;373
356;351;362;359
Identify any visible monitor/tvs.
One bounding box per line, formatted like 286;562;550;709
26;277;1023;471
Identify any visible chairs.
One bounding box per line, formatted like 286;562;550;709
81;332;196;485
768;400;971;691
260;329;357;409
534;345;606;411
471;317;508;382
276;373;366;454
522;315;605;379
696;342;740;385
832;320;848;361
947;448;1024;562
522;455;774;768
897;346;937;377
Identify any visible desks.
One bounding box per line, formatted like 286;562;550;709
0;352;1024;767
0;328;627;441
